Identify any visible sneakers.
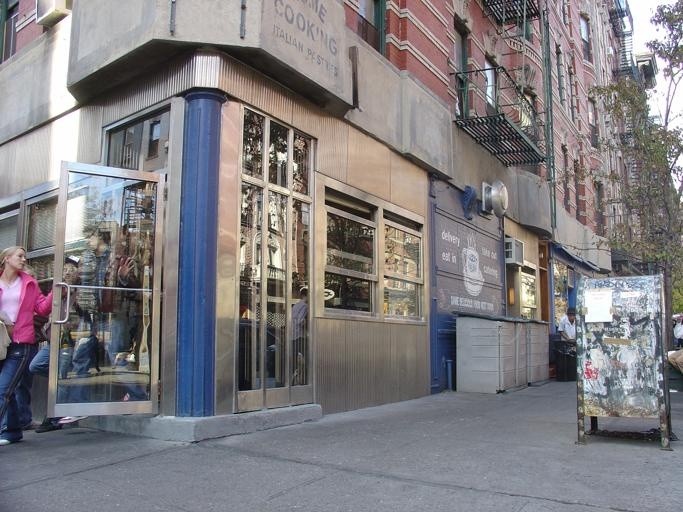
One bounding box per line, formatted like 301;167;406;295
36;416;88;432
0;431;22;445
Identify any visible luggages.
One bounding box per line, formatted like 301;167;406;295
555;339;576;382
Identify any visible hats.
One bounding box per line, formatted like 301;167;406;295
566;308;575;314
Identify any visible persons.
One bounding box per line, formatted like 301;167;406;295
287;288;311;385
671;316;683;347
558;308;579;341
0;226;148;446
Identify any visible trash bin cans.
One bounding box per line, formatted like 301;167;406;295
552;340;576;381
